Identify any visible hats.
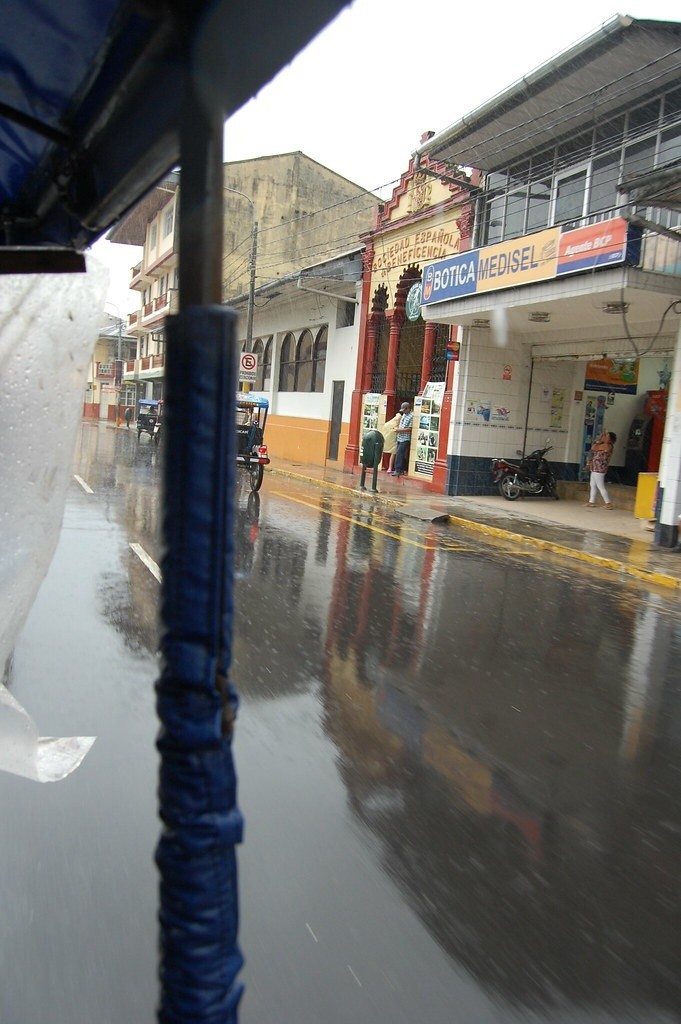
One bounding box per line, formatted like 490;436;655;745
399;402;410;411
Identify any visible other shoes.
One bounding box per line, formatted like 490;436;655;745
392;471;404;476
387;468;393;473
584;502;596;507
600;502;614;510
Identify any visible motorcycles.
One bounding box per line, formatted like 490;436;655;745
490;435;558;500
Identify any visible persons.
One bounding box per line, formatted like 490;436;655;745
149;406;156;413
125;407;132;429
244;408;255;426
583;432;617;510
382;402;414;476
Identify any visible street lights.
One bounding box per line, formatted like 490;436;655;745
171;169;257;392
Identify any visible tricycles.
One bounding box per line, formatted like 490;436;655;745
235;392;271;491
137;399;162;444
